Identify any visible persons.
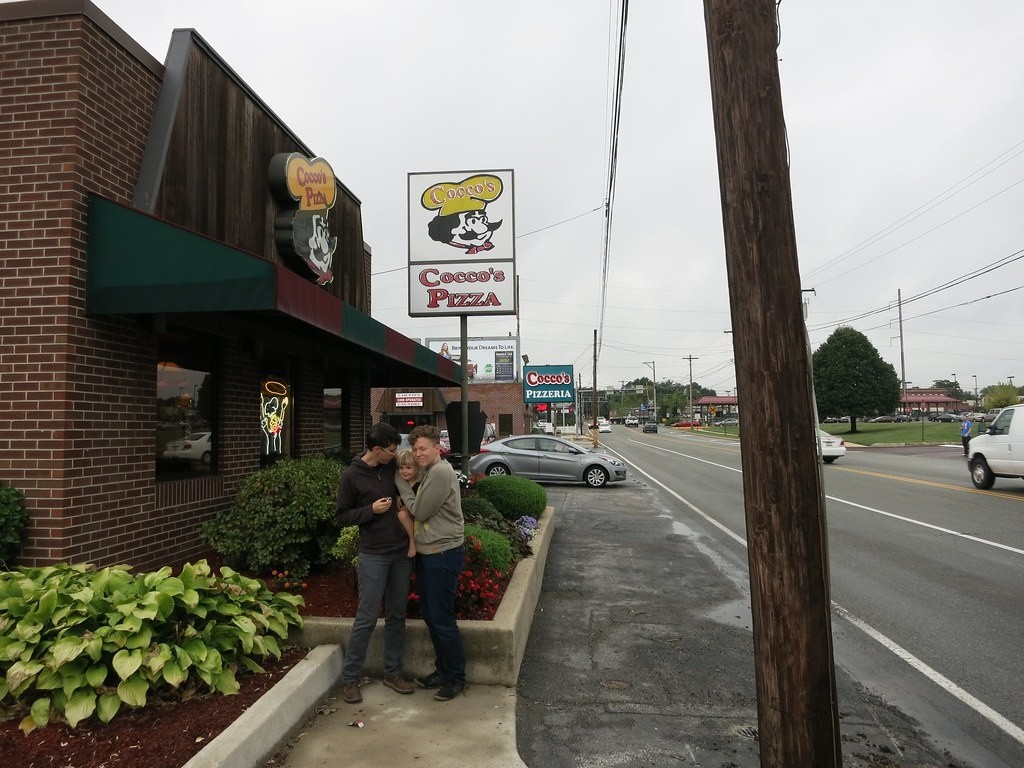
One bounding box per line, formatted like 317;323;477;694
395;449;422;557
960;415;972;455
394;425;466;701
336;422;415;704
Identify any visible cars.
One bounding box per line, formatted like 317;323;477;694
815;429;846;464
625;416;639;427
162;432;212;465
967;413;986;423
598;422;612;433
929;412;971;422
824;416;848;423
468;433;627;489
439;430;471;467
716;419;739;426
981;408;1004;423
869;414;913;423
480;422;496;446
597;416;606;423
642;421;658;434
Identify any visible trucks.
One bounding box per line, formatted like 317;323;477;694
968;404;1024;490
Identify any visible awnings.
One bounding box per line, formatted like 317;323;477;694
86;192;465;386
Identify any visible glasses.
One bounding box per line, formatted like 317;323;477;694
374;446;397;458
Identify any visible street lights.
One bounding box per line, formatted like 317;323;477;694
972;375;977;413
952;374;957;409
1007;376;1014;381
662;376;693;430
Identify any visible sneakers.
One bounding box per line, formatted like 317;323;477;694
432;682;464;701
343;680;362;702
383;675;415;694
414;672;440;688
